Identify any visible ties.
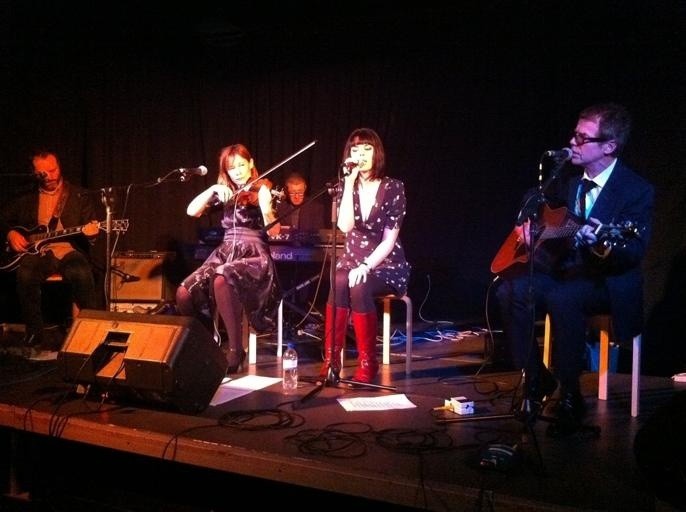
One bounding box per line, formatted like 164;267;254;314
579;179;596;219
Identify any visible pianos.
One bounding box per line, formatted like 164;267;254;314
195;227;347;264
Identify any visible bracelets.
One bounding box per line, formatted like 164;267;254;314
362;262;372;273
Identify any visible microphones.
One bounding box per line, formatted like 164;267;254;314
547;147;573;160
180;165;208;178
39;171;49;179
343;163;357;175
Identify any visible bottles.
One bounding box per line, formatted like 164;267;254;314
282;343;298;392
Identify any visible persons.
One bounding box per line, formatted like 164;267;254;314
496;102;655;422
275;173;325;299
176;144;281;374
0;151;106;350
318;129;411;389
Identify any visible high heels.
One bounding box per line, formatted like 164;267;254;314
224;347;246;375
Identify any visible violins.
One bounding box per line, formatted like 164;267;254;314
235;177;286;206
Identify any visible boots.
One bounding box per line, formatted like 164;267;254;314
348;309;379;388
315;302;351;388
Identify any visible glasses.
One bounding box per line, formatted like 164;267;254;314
571;127;611;146
288;190;305;197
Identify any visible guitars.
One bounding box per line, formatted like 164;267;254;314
0;220;129;272
491;203;645;277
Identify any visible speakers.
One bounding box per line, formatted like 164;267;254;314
55;306;229;415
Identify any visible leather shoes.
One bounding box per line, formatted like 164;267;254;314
513;369;558;424
18;330;44;347
545;390;590;439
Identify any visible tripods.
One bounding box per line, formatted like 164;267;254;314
292;197;398;410
436;219;601;472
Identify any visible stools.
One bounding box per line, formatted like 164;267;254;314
542;312;641;417
213;296;283;365
340;290;412;368
